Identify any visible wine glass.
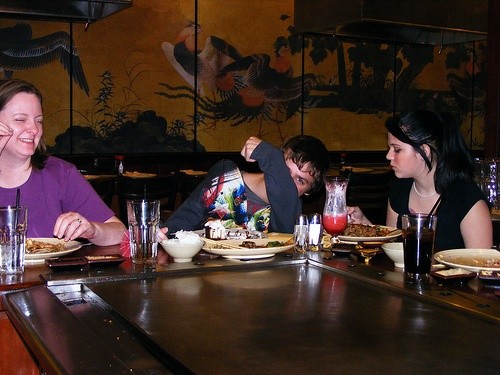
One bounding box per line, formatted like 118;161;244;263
322;176;350;255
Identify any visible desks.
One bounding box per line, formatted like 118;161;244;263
1;244;500;375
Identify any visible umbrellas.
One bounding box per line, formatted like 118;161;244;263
120;229;132;258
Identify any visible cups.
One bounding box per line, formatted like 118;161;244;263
294;214;309;251
125;199;160;265
402;213;438;284
308;213;322;252
0;205;27;274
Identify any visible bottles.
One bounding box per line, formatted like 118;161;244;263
474;156;500;210
206;213;216;240
113;156;127;174
338;153;349;166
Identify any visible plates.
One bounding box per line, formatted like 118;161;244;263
433;248;500;276
433;266;477;286
85;170;210;180
340;168;374;174
43;253;125;271
323;223;402;245
25;236;83;266
202;238;298;260
476;269;500;286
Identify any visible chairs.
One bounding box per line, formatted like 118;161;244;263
88;169;394;226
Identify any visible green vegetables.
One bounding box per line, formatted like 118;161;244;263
268;241;282;247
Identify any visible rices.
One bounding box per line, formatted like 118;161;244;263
162;230;203;247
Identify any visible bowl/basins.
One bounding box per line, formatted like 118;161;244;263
381;243;404;267
160;239;205;263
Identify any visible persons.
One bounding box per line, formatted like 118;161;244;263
0;79;128;246
385;109;493;251
157;132;328;239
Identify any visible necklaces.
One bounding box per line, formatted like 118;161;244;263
413;182;436;198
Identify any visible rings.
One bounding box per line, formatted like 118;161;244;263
76;218;82;225
